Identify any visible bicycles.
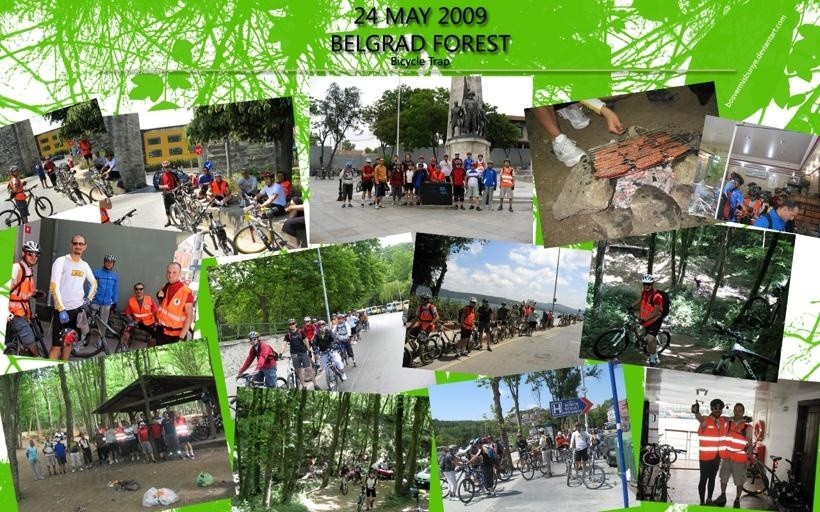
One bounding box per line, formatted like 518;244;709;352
195;409;223;439
162;173;297;258
53;165;130;207
696;283;790;381
639;443;685;502
339;465;361;496
441;425;609;504
70;298;137;357
356;482;368;512
4;289;50;361
109;209;139;226
403;302;583;370
742;451;810;512
693;183;717;217
592;303;673;361
317;166;390;192
231;321;371;416
0;183;54;229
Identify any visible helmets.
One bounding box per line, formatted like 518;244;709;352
22;241;41;252
162;160;275;179
447;421;581;450
642;274;655;283
104;255;116;262
419;293;535;310
247;309;365;339
58;328;78;343
9;151;113;176
346;152;511;170
730;172;791;206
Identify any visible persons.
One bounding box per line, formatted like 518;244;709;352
532;94;631;168
0;132;802;511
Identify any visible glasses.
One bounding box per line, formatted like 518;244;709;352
135;288;144;290
29;253;40;257
72;243;85;246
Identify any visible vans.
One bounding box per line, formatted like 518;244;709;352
357;300;409;315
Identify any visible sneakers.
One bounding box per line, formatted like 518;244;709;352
552;134;587;167
648;91;680;104
33;451;195;480
342;195;513;212
413;345;492;362
302;360;356;391
700;494;740;508
650;354;656;366
556;107;592;130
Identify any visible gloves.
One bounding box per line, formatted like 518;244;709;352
59;311;70;324
33;291;46;299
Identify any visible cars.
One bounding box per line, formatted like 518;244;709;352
416;465;430;491
377;465;396;481
154;170;193;191
56;432;68;438
601;434;630;468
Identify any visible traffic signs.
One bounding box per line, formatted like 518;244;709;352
548;396;594;418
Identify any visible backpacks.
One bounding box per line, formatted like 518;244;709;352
650;289;669;317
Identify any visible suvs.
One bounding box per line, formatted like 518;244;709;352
437;445;448;462
